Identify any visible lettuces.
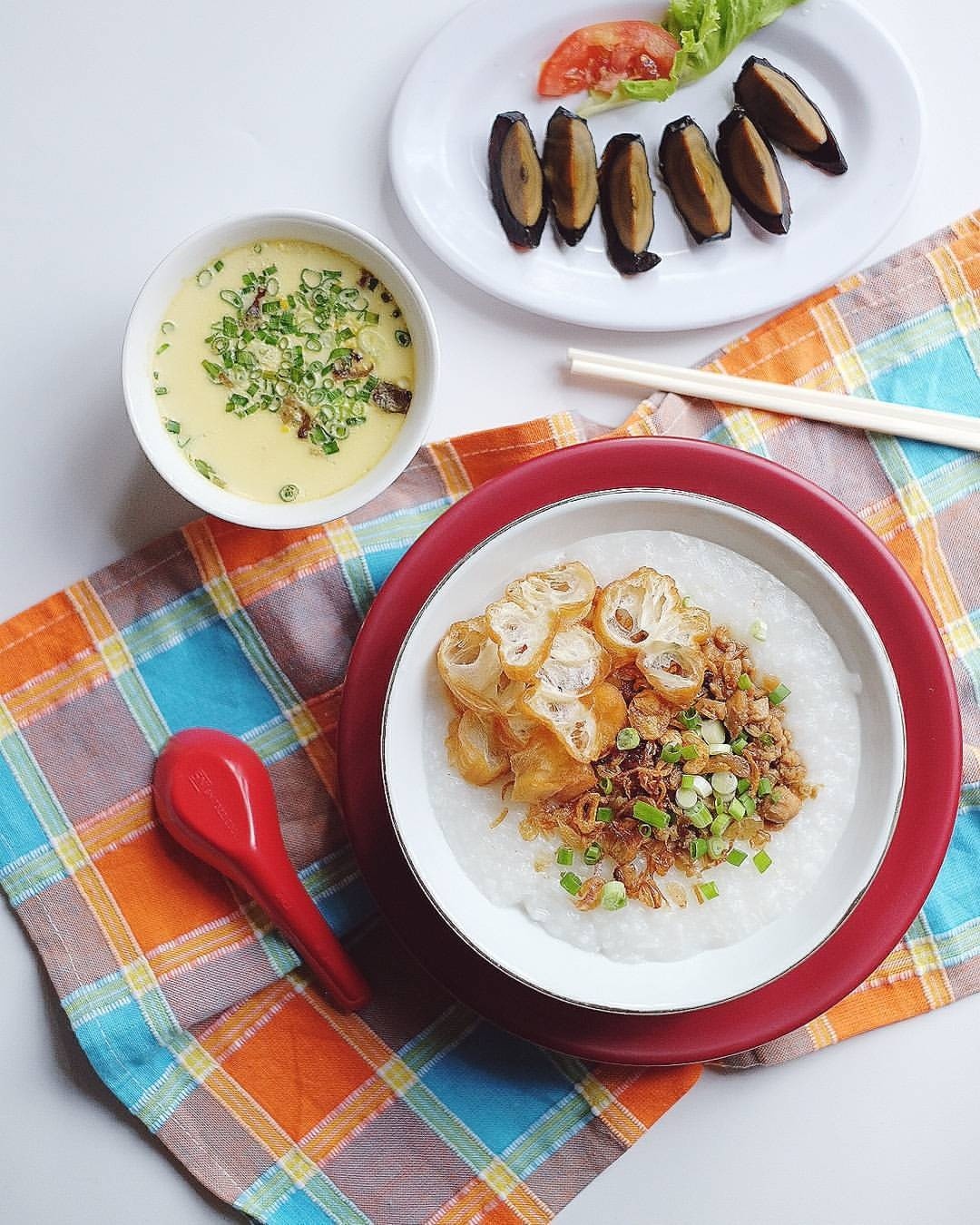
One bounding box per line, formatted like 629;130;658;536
578;0;810;117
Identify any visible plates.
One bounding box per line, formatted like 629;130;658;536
334;435;962;1065
383;1;925;332
378;484;910;1017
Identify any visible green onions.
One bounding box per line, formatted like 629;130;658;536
557;619;791;912
154;244;412;505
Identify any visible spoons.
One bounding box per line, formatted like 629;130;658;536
155;729;375;1019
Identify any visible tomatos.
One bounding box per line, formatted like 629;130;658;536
538;20;681;97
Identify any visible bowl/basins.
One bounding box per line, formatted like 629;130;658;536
119;207;441;532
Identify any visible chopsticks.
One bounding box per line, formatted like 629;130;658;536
567;348;980;455
